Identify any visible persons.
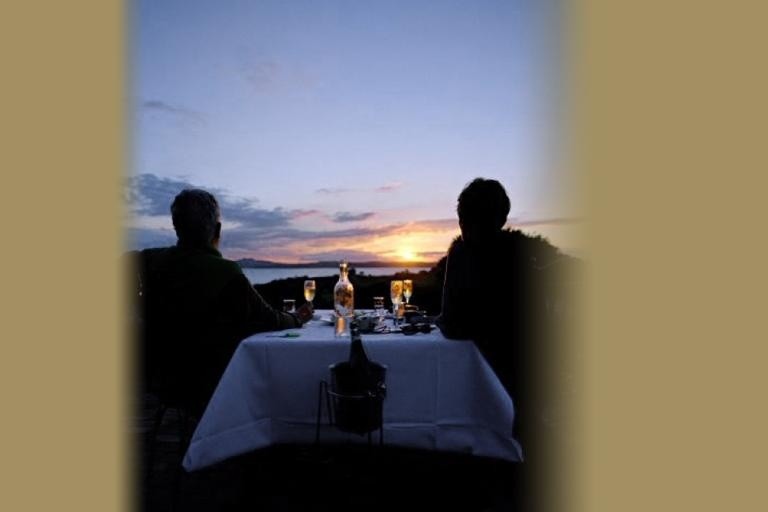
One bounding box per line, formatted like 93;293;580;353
407;176;580;400
138;188;315;413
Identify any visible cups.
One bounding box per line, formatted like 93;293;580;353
283;299;297;315
373;297;385;319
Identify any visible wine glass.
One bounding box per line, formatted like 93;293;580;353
390;280;405;331
403;279;413;304
304;279;316;315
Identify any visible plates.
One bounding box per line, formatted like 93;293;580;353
320;313;367;326
395;322;437;331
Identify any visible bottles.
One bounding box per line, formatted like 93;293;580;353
331;260;357;338
347;320;371;374
